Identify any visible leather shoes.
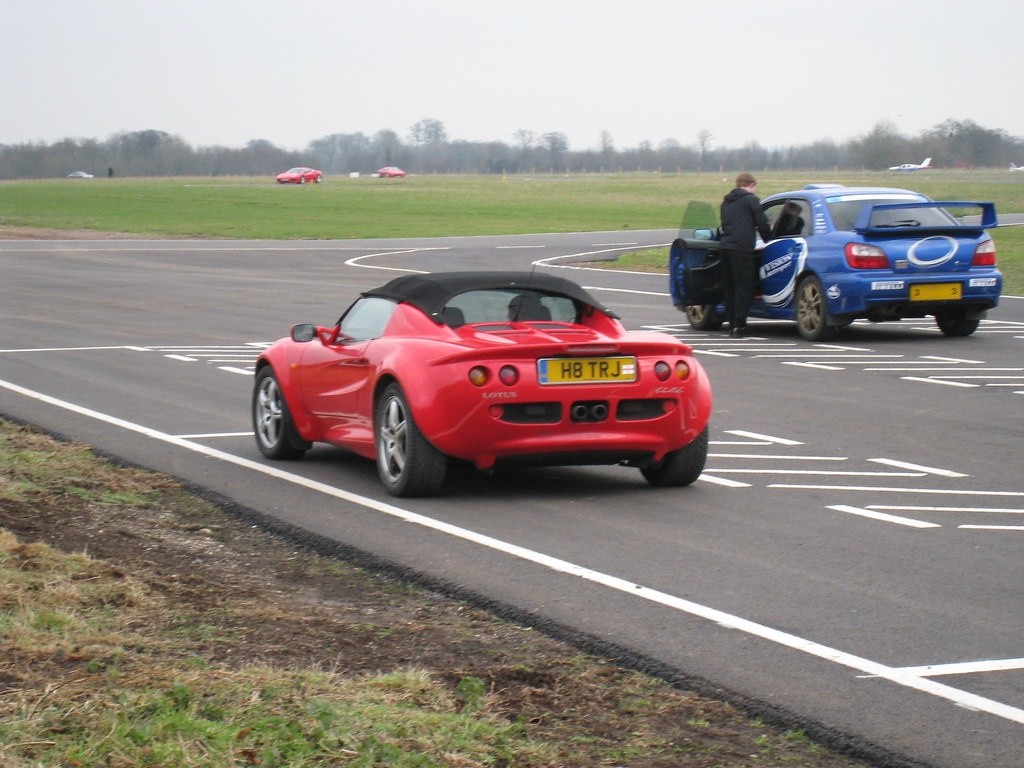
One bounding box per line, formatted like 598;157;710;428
729;325;761;338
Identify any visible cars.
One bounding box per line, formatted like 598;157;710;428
67;171;94;180
378;167;405;178
668;184;1005;342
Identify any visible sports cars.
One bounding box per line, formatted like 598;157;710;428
250;271;714;497
278;168;324;187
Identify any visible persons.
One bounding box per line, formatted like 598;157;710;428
773;202;805;242
719;173;772;338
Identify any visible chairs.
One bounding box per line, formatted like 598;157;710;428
443;308;465;328
523;305;551;322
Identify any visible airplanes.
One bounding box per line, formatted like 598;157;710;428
889;158;933;172
